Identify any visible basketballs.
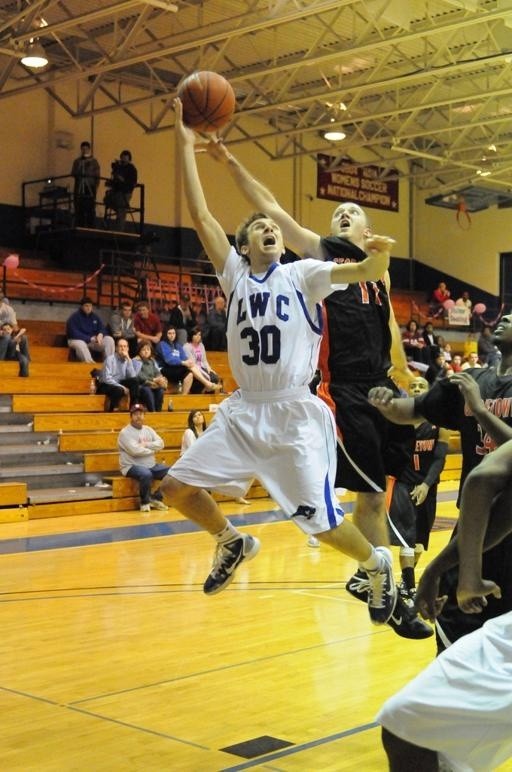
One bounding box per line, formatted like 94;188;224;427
178;71;234;131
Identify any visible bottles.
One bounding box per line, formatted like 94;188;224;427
217;377;224;394
177;379;182;395
88;377;97;395
168;397;173;412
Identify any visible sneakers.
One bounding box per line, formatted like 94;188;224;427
347;548;434;640
150;499;169;511
140;503;150;512
203;532;261;596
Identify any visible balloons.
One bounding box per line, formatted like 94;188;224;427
443;299;456;310
475;303;486;313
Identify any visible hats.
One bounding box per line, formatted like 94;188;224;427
130;404;145;413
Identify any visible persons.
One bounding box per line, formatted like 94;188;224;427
207;297;228;352
159;98;400;624
132;301;164;356
461;352;481;370
97;338;142;412
0;291;32;377
367;315;511;771
72;141;100;223
182;328;219;395
180;410;251;504
105;150;138;231
132;340;168;412
155;324;222;394
196;117;437;642
118;404;172;512
66;296;116;363
167;293;200;347
105;301;141;360
387;376;449;605
428;282;450;320
477;326;502;368
464;333;477;360
402;320;432;366
456;292;473;321
450;353;465;372
425;352;451;389
422;322;453;364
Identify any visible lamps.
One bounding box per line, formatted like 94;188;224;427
324;104;347;140
21;22;48;68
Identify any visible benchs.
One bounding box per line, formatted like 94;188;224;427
0;320;269;523
439;431;463;482
